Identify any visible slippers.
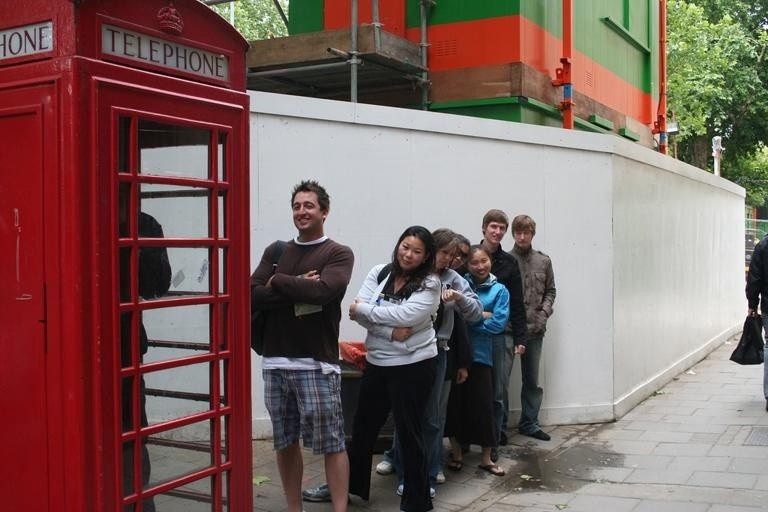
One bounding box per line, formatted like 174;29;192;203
448;454;464;470
479;462;504;476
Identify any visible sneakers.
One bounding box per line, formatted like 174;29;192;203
302;482;332;501
430;487;435;497
436;472;445;483
376;461;392;475
398;484;405;495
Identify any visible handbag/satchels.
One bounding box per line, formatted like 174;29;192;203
251;240;289;357
730;313;766;365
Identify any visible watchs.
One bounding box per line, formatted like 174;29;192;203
455;289;462;301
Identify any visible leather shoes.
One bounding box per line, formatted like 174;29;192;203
462;444;471;454
500;433;507;445
520;426;551;441
490;450;498;463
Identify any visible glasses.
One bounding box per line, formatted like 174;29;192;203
454;247;468;263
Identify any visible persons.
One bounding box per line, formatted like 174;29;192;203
445;244;505;478
391;227;483;500
745;233;767;391
457;209;528;462
376;233;468;484
118;153;171;511
497;214;557;444
251;181;352;511
302;226;442;512
